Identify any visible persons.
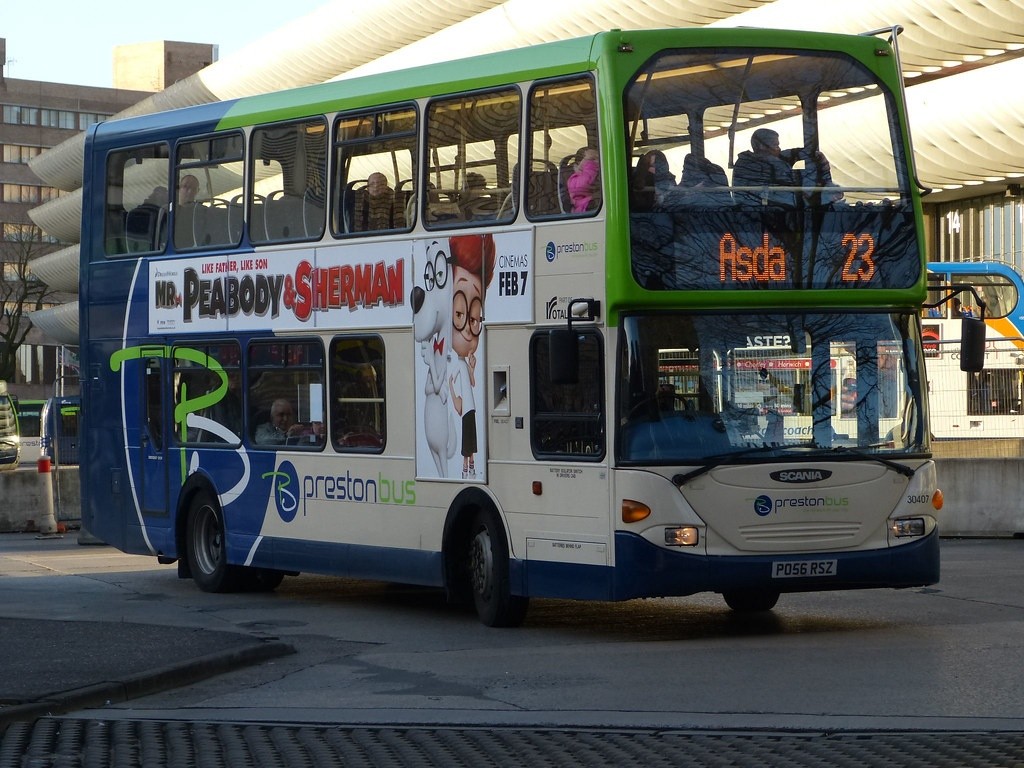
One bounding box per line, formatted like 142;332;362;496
342;362;377;429
360;173;394;230
126;187;167;245
297;411;354;446
737;128;843;204
954;297;965;317
567;147;602;214
255;399;304;445
176;175;200;248
459;172;488;191
631;149;732;205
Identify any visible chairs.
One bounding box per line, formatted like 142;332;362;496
926;306;973;318
126;155;576;253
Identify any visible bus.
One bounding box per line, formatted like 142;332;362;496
80;25;987;631
656;263;1024;447
0;381;81;470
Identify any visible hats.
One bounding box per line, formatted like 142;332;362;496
953;297;961;306
